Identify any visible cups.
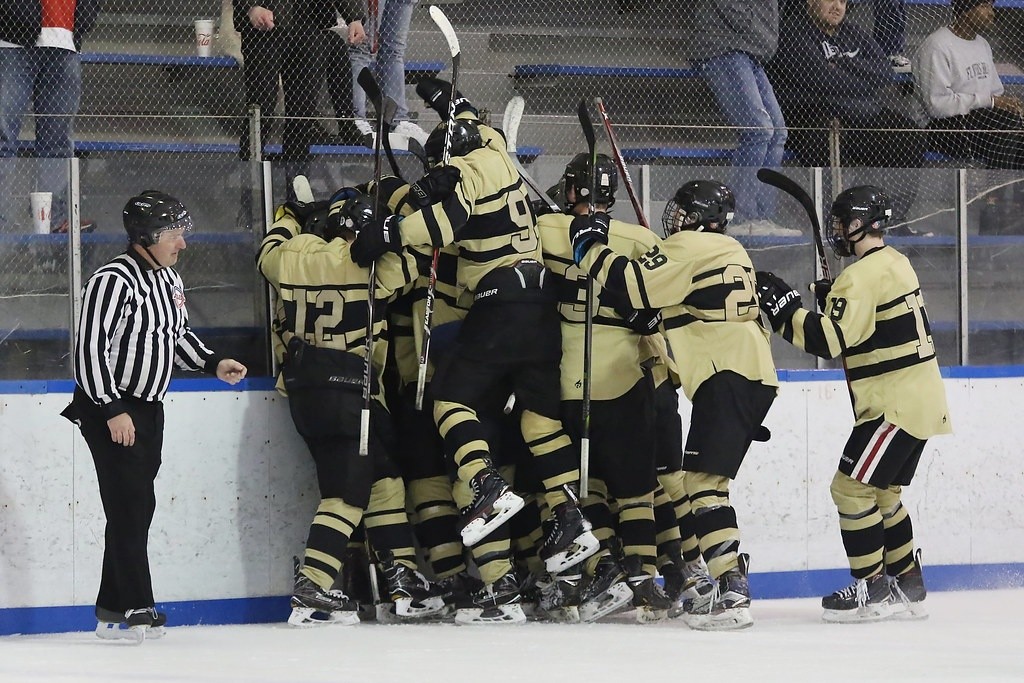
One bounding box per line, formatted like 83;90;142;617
194;19;214;59
30;192;53;235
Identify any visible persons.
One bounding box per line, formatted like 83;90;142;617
753;185;953;622
231;0;429;202
686;0;1024;237
570;179;780;631
255;78;715;627
0;0;99;236
58;189;248;645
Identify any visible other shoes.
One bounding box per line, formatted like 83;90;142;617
979;202;1024;235
897;224;935;240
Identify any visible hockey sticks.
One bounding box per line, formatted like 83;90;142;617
502;94;561;214
291;172;316;205
755;167;859;423
360;131;432;174
357;65;388;457
413;4;466;413
593;97;773;444
573;95;598;500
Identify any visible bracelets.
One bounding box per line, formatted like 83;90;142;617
991;97;995;108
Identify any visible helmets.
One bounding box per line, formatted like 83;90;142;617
424;119;481;167
122;189;187;248
336;195;393;233
563;152;618;205
661;180;736;238
826;182;892;256
301;209;336;242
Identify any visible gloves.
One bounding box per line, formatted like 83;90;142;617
407;166;460;208
569;211;610;268
349;211;406;269
329;199;353;229
754;270;803;332
615;303;663;335
807;278;834;314
417;76;478;125
283;193;318;226
330;185;362;206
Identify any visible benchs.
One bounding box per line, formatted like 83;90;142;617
0;0;1024;338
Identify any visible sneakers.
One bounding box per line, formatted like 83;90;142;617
459;454;522;547
539;481;599;574
95;599;164;645
823;546;893;621
286;536;756;632
887;547;929;618
